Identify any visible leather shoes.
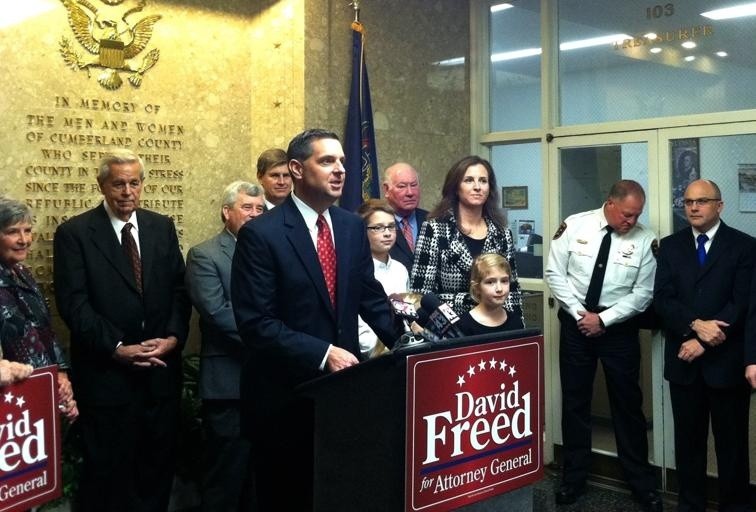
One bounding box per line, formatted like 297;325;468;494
555;469;584;502
632;484;663;509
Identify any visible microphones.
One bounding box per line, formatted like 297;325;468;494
420;292;465;339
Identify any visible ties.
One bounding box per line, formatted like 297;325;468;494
316;214;338;309
693;230;713;265
120;221;146;294
584;222;619;311
399;217;416;253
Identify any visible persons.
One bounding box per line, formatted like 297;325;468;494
409;155;525;330
455;252;524;337
187;180;265;512
1;200;79;425
653;180;756;511
357;199;411;360
257;150;292;213
232;128;407;512
382;162;432;277
545;180;663;512
0;347;34;385
54;149;192;512
744;299;756;389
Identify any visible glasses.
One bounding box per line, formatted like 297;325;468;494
682;196;719;204
365;223;397;231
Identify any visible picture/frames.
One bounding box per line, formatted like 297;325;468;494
502;186;528;210
